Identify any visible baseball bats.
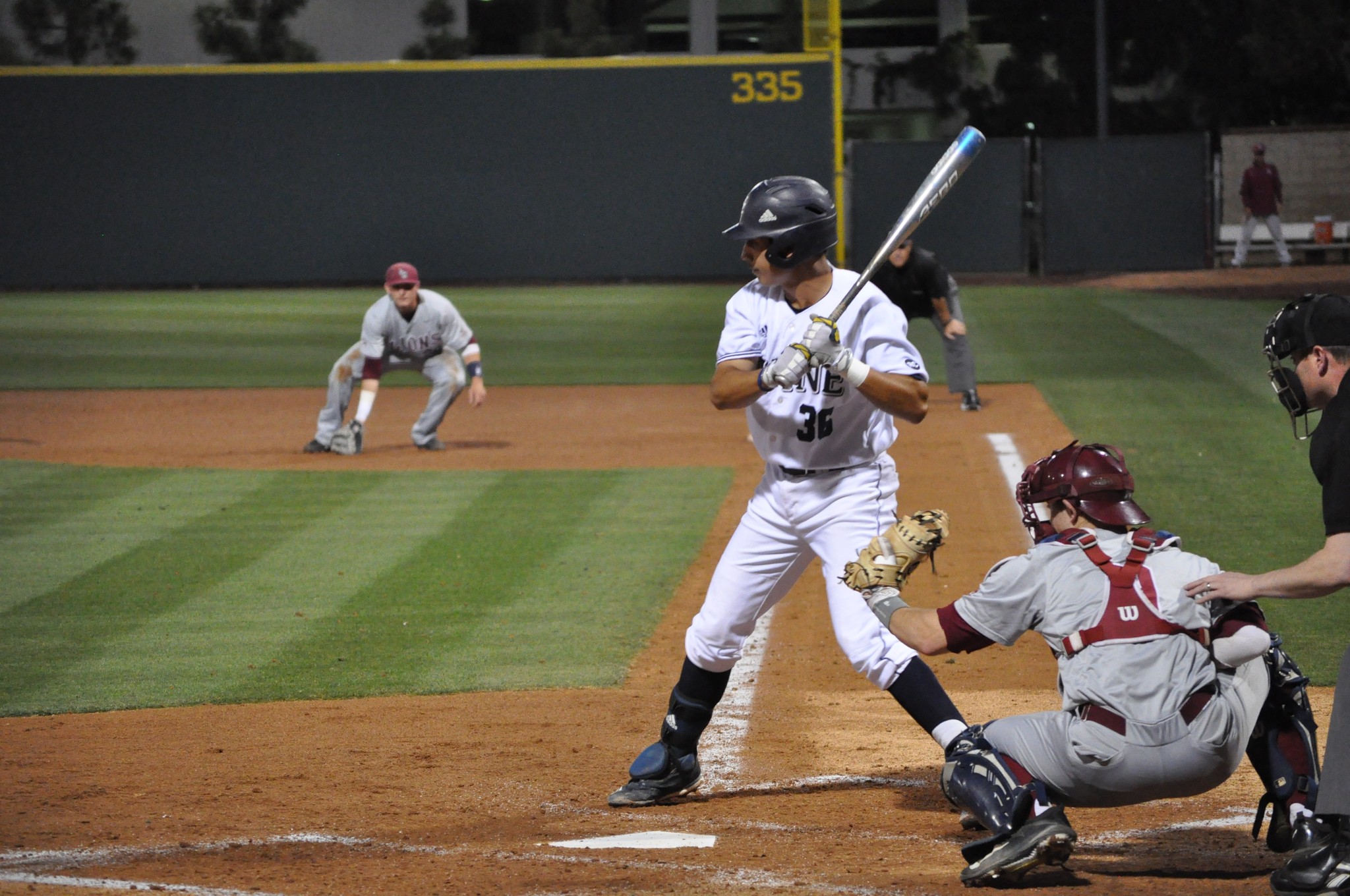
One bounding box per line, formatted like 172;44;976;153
774;122;989;389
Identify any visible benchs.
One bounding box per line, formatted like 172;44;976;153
1213;222;1350;269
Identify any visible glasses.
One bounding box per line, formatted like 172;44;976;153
898;242;912;250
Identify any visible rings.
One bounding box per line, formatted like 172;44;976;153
1207;582;1210;590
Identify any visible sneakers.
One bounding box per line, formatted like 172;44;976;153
1288;811;1331;851
959;810;1077;888
302;439;327;454
959;389;981;411
1270;816;1350;896
607;754;702;808
419;439;445;450
960;809;985;832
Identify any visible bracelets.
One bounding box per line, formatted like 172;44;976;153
467;362;482;378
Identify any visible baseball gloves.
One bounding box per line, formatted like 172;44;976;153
329;418;365;456
836;506;951;592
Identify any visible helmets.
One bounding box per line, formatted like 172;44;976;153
1042;444;1152;525
721;175;836;269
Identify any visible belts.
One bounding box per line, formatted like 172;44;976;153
1078;684;1216;737
779;465;843;478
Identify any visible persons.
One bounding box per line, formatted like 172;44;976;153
401;0;468;61
191;3;319;64
1183;289;1350;896
303;262;486;455
607;177;983;829
869;232;982;412
844;440;1322;885
1230;142;1293;267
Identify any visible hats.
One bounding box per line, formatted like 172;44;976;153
1275;293;1349;361
385;263;419;286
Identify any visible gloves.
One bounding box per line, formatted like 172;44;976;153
800;313;850;375
761;341;814;392
